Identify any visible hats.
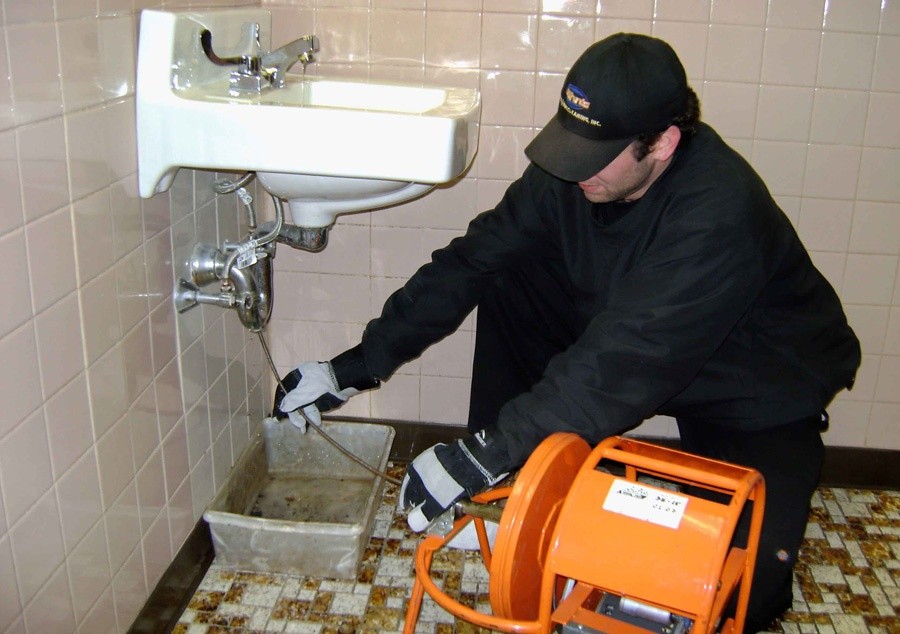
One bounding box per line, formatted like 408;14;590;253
523;32;687;182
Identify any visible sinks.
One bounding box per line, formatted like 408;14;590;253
135;5;483;228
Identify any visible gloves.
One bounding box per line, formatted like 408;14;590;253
274;361;360;435
400;426;509;531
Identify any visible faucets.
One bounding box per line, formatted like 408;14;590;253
261;35;320;89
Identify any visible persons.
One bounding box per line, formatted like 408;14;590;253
272;33;862;634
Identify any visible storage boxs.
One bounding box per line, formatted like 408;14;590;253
202;415;398;582
